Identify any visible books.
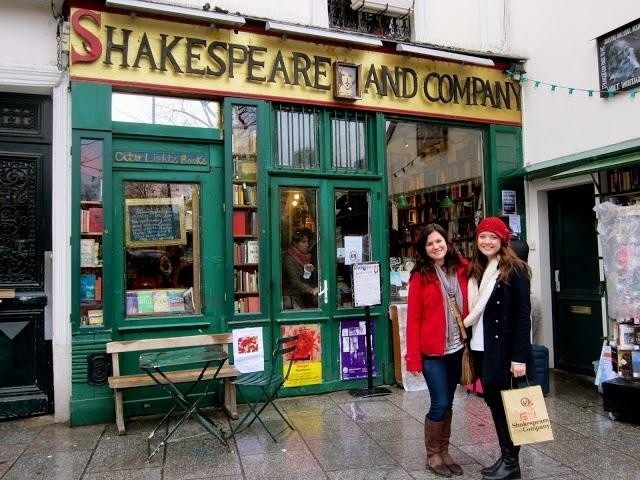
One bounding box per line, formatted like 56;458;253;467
79;205;105;326
231;126;258;316
123;287;196;315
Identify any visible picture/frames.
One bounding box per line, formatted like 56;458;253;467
333;61;362;100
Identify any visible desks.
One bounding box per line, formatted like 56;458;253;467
138;343;233;464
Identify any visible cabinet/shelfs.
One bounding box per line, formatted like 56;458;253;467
386;177;482;260
81;200;103;326
232;125;260;314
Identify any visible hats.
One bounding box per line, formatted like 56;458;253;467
474;216;512;247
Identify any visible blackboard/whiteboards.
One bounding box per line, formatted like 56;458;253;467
124;197;187;248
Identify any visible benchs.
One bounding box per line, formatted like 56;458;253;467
106;333;241;436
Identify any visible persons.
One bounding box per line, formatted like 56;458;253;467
461;215;535;479
606;37;640;85
401;221;472;477
281;227;320;310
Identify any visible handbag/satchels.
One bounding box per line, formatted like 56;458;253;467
500;375;554;447
460;346;475;384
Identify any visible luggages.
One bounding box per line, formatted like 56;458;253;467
516;346;549;396
463;378;483;395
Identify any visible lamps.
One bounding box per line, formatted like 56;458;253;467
266;20;383;48
106;0;246;27
396;43;495;67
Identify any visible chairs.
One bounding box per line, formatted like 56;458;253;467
224;335;298;443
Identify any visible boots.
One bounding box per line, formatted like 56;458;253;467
424;417;452;477
481;447;504;475
481;447;522;480
441;416;463;476
